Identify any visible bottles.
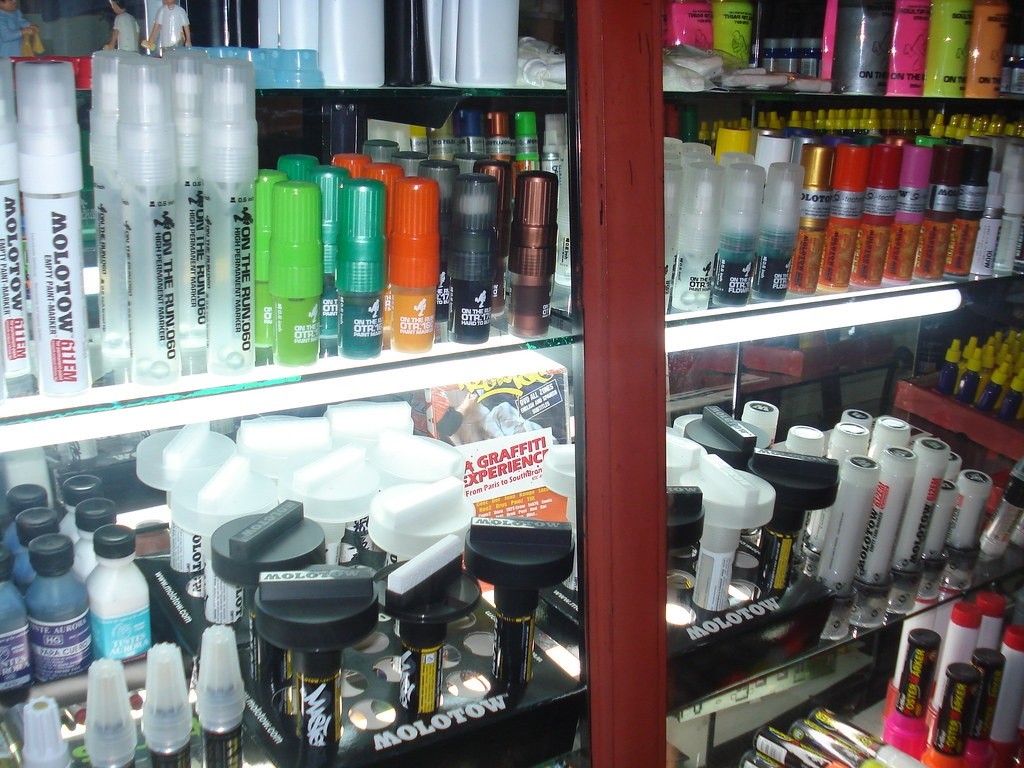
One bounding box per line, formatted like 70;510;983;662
0;475;152;707
761;37;821;79
1;48;257;401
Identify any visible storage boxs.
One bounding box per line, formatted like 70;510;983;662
673;324;892;377
45;412;257;516
894;369;1023;460
532;538;836;708
365;348;573;446
133;552;588;768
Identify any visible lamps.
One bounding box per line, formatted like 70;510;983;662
664;279;961;350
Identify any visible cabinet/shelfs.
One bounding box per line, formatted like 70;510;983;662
1;0;1024;767
